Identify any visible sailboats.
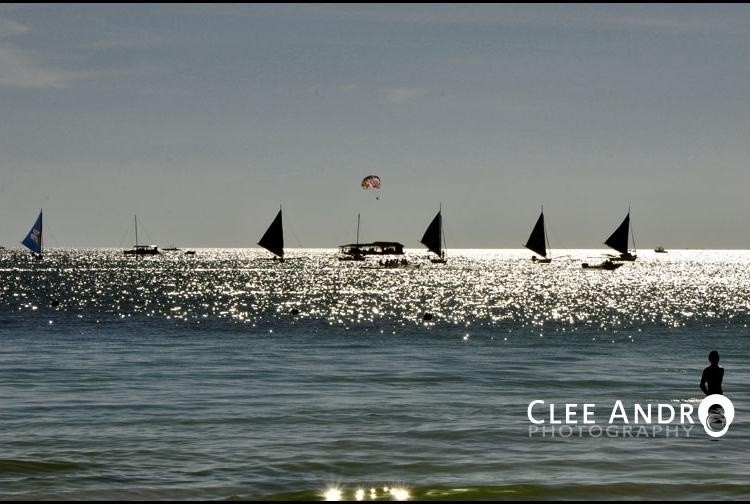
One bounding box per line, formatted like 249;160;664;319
256;204;301;261
20;207;45;260
580;202;637;262
521;203;551;264
417;202;448;264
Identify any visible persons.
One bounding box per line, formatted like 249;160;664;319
352;244;403;252
700;350;724;409
378;256;411;267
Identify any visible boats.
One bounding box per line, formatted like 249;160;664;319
582;260;624;270
337;212;421;269
123;215;196;255
654;246;668;254
